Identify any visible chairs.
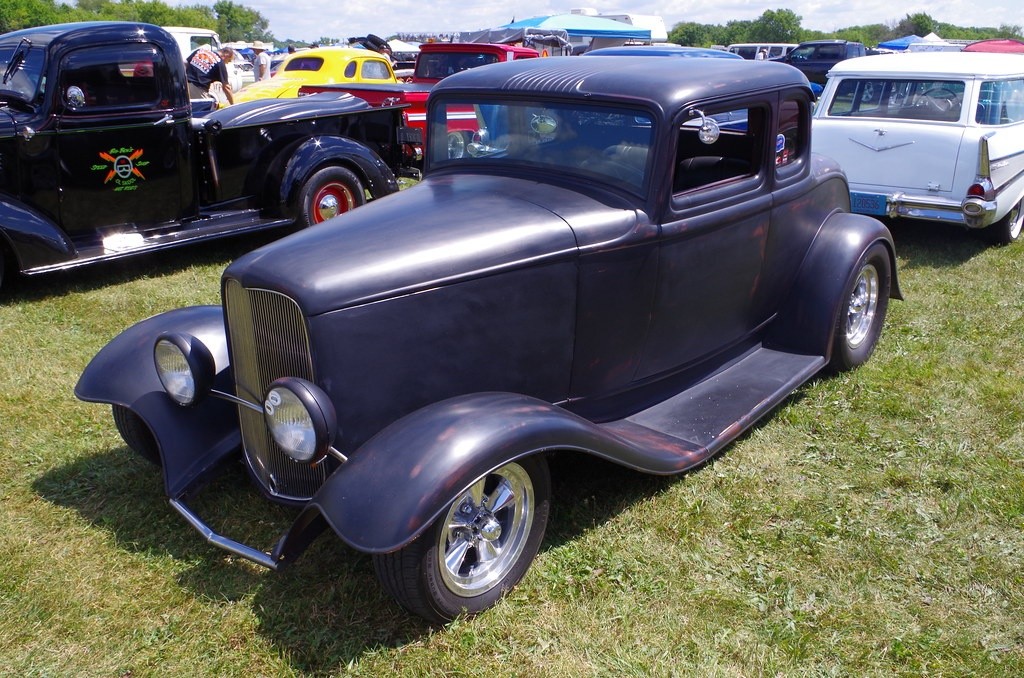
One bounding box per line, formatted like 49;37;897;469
95;81;160;109
898;105;939;121
678;156;748;194
979;90;1009;125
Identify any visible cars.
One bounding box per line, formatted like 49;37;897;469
807;46;1023;244
154;19;904;172
71;52;904;631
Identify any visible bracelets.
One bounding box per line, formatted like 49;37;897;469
258;77;262;80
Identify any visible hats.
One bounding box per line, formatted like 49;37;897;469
308;42;319;48
247;40;266;51
761;49;768;53
288;45;296;52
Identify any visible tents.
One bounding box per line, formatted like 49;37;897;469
878;31;1024;55
500;13;651;57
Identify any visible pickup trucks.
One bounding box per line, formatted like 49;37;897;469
0;19;422;306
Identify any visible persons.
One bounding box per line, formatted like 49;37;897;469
183;47;234;111
756;49;768;61
249;40;271;82
288;44;296;54
309;43;320;49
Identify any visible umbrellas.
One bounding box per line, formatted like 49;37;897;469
240;45;287;60
